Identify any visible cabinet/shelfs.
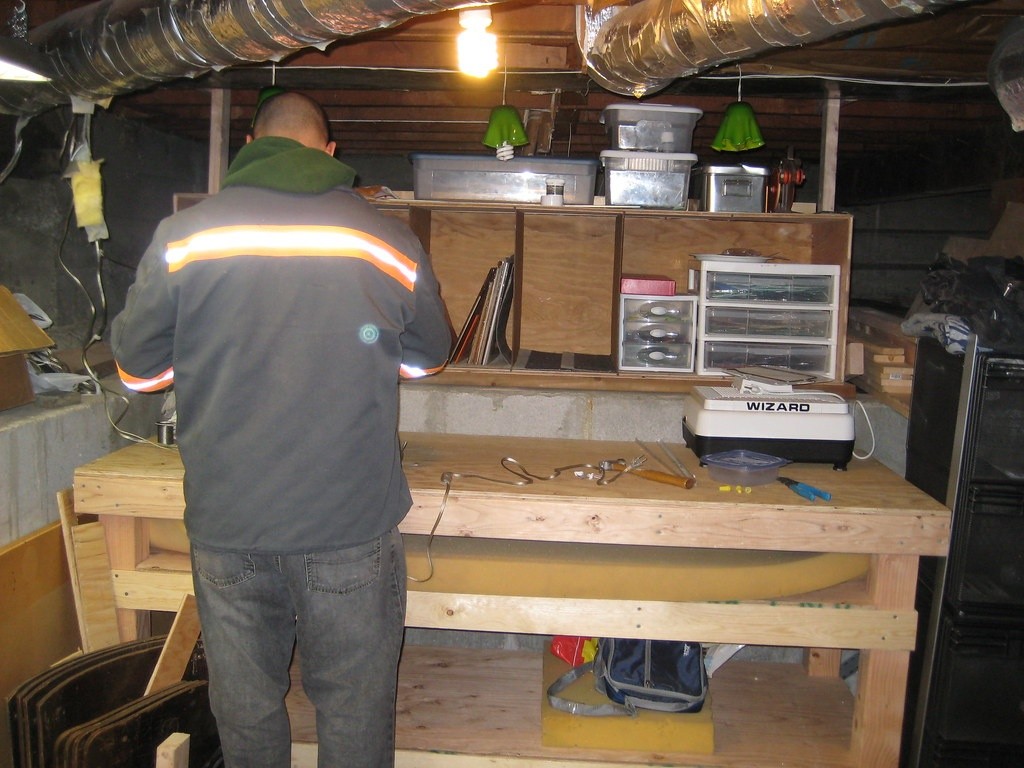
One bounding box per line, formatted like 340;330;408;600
618;293;698;373
368;199;853;386
688;259;841;382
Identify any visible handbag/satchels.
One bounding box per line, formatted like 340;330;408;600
547;638;709;717
13;293;98;395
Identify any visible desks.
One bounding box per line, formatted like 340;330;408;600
72;428;953;768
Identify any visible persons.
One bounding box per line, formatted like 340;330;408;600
107;90;454;768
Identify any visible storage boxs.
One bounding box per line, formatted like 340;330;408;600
408;154;602;205
692;166;769;212
0;284;55;412
620;272;676;295
600;104;704;211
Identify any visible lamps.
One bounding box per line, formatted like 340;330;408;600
711;64;765;152
456;5;498;80
0;38;56;84
482;54;529;162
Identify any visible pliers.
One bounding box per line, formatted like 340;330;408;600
776;476;832;502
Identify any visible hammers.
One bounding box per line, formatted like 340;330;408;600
599;458;694;490
